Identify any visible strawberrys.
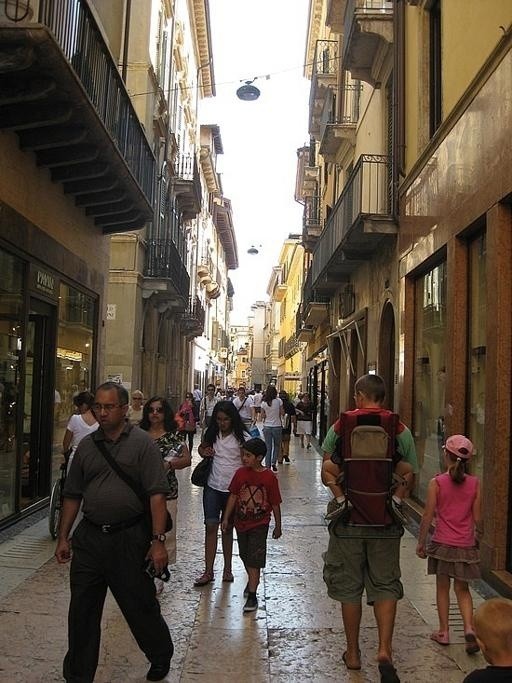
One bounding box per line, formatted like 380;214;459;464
47;447;72;539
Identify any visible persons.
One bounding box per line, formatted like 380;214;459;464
293;393;314;449
178;392;199;459
125;390;144;425
193;401;251;586
221;438;283;611
463;598;511;683
199;384;220;442
139;396;191;565
216;387;263;426
62;392;100;540
193;384;203;417
320;376;419;682
56;381;174;683
278;390;296;464
321;439;413;524
261;385;285;471
415;434;481;652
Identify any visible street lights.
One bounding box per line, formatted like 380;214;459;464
173;411;186;431
281;412;290;429
199;410;206;428
191;443;214;487
184;413;195;432
92;431;174;534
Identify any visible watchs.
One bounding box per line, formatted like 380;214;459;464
150;534;166;543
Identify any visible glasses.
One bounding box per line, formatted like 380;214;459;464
247;245;258;254
233;76;268;101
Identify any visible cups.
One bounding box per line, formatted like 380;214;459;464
208;389;213;391
90;404;121;411
148;406;166;413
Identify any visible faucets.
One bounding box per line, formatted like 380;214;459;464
194;572;215;586
222;571;234;582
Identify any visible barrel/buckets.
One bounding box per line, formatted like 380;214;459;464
342;648;362;670
378;661;401;683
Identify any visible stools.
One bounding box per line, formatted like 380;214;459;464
82;520;140;535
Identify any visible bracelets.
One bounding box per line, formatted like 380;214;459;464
168;461;172;470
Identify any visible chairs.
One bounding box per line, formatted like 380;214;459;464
272;463;278;471
324;494;352;520
431;630;450;646
387;497;410;525
243;599;258;611
464;629;481;655
244;580;259;596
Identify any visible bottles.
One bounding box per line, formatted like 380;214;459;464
442;434;474;460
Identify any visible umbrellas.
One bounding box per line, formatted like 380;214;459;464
284;455;290;462
147;659;171;682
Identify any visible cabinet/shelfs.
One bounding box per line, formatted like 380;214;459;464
164;444;184;464
